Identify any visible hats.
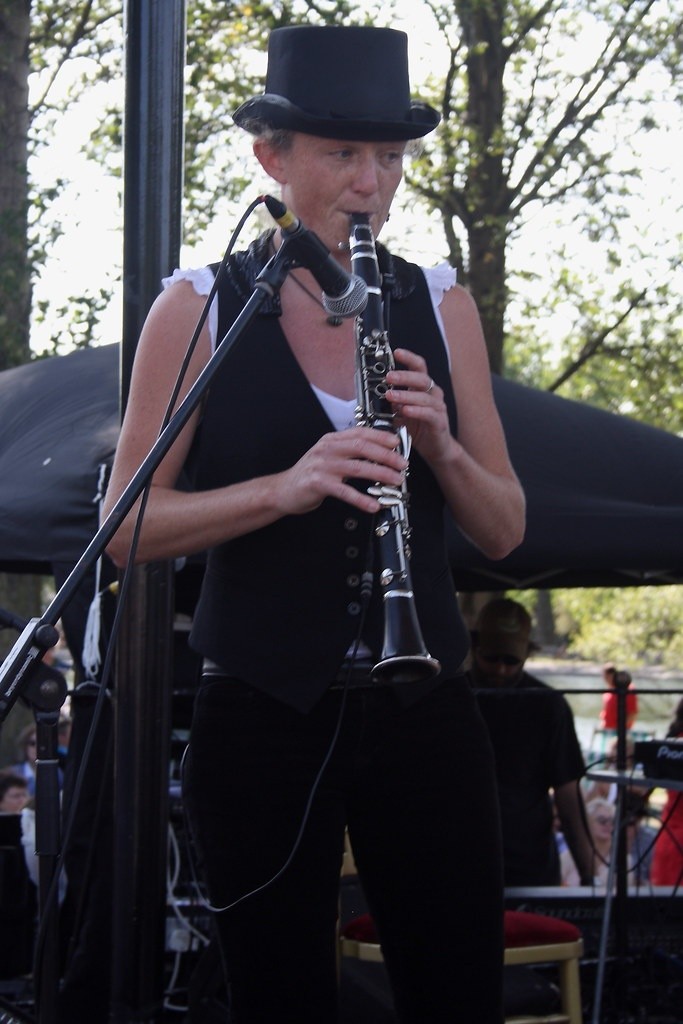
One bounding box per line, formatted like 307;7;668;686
475;598;532;661
232;23;443;141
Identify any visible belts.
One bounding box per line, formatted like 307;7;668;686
202;656;376;689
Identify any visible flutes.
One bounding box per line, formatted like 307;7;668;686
346;211;442;686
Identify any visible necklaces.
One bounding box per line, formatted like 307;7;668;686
270;234;343;327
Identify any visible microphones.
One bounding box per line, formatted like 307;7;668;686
264;195;369;319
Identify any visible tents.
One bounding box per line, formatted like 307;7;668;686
0;341;683;620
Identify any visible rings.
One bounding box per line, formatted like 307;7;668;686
425;379;434;393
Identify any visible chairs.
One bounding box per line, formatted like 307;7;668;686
325;822;599;1024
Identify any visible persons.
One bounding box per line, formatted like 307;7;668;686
0;616;74;980
100;22;526;1024
461;597;683;887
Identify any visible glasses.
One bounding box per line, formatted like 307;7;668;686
27;740;35;745
